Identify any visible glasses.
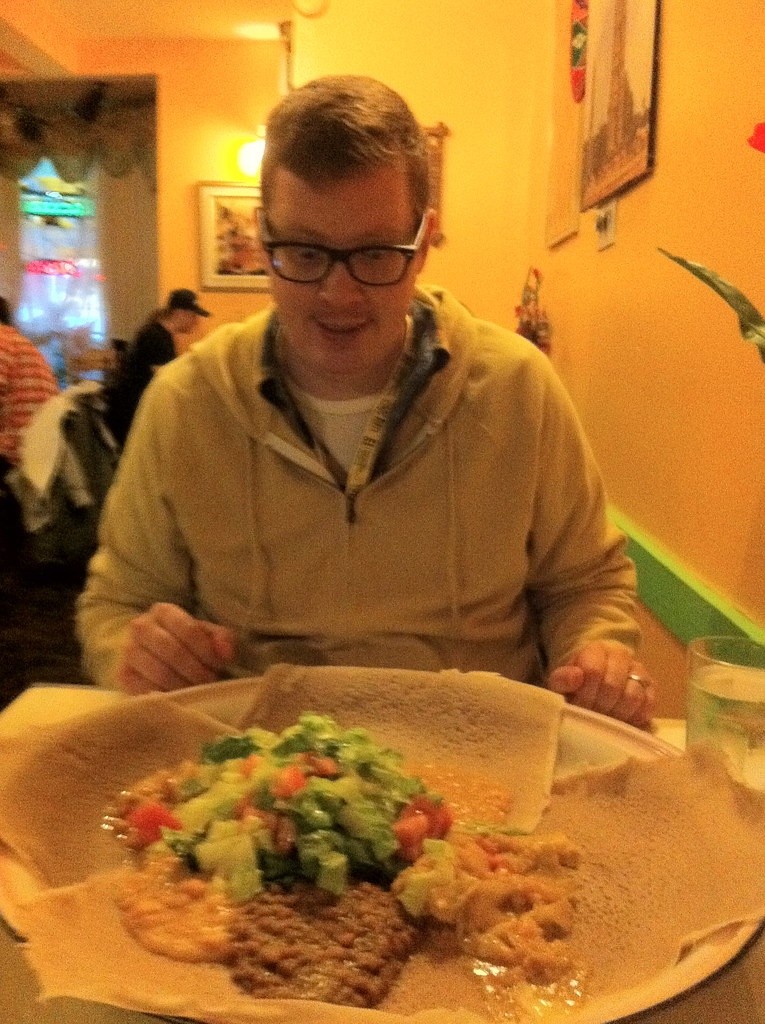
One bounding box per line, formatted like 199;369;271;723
261;213;431;286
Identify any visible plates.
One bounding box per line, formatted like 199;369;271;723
0;677;765;1024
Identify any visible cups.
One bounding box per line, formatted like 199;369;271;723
686;635;765;803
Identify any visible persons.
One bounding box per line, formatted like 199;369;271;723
78;74;662;731
0;298;59;490
107;288;209;449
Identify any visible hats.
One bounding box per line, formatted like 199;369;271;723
171;289;210;317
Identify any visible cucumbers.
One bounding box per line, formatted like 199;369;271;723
163;711;427;893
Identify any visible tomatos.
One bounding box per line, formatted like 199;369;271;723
125;801;184;847
393;794;452;853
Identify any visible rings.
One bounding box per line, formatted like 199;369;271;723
628;675;647;688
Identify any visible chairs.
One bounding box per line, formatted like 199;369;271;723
1;379;100;589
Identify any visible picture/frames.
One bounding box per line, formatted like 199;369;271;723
193;181;271;293
